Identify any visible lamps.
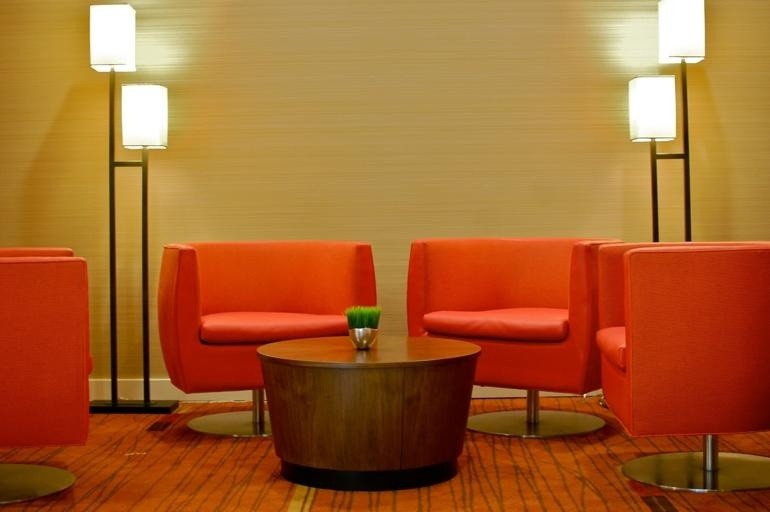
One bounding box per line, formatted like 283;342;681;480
86;6;179;413
626;0;705;241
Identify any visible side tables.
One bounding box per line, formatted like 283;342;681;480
255;335;483;481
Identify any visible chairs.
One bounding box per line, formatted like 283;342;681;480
405;240;770;494
2;246;95;506
159;241;380;439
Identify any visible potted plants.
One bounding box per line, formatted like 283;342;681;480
346;307;380;353
